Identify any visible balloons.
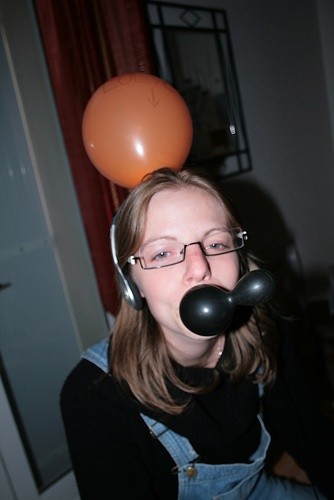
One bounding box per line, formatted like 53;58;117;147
180;269;281;336
82;73;193;188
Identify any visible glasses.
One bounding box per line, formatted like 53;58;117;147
127;227;248;270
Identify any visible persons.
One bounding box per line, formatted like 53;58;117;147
59;168;327;500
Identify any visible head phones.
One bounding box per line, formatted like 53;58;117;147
111;201;143;311
